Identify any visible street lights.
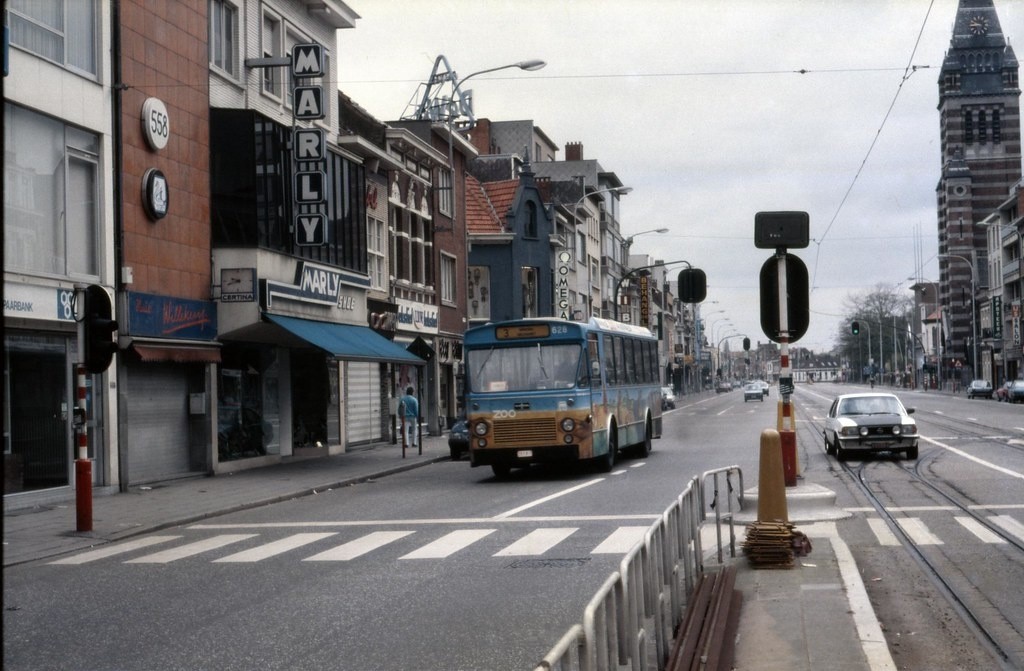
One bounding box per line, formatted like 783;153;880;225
979;222;1024;349
569;182;742;392
936;253;978;379
908;277;941;390
448;57;547;231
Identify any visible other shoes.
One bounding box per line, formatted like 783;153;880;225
404;445;409;448
412;443;417;447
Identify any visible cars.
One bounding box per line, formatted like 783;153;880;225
997;379;1024;403
744;384;764;402
754;380;769;396
715;382;733;393
660;387;675;409
733;381;740;388
822;392;919;462
967;380;993;399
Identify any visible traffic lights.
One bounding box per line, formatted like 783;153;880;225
83;284;123;376
853;322;859;335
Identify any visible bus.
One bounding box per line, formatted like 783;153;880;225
463;317;669;478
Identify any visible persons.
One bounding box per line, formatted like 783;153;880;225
870;370;876;388
398;387;419;448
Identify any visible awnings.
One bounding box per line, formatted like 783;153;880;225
262;312;427;364
